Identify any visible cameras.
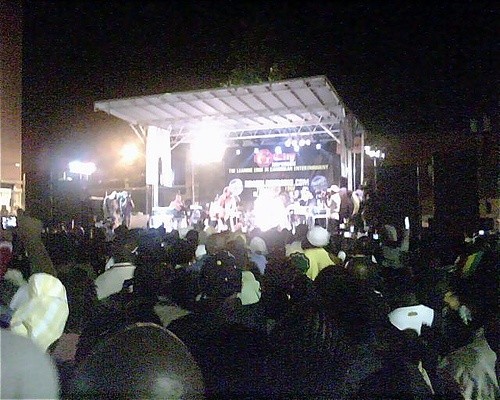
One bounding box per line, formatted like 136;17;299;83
2;215;18;231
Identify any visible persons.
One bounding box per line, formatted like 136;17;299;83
0;185;500;400
